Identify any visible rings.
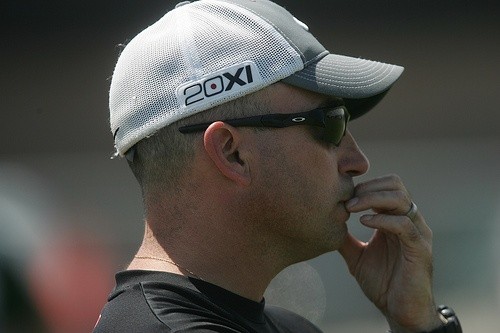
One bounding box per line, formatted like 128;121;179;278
405;201;418;220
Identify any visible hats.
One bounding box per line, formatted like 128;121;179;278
109;0;406;158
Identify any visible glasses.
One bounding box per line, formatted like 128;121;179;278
179;99;351;150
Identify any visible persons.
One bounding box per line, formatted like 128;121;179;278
93;0;464;333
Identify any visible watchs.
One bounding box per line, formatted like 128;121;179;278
422;304;463;333
